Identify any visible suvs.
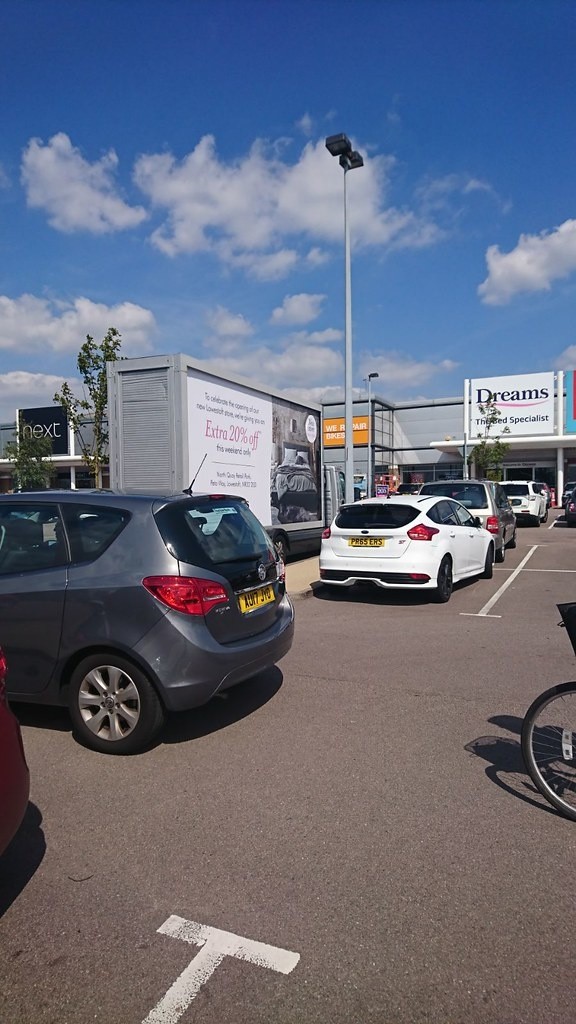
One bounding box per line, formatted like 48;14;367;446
498;481;548;527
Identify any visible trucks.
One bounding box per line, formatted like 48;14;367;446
106;352;345;566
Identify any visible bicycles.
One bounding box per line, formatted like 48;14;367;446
522;601;576;822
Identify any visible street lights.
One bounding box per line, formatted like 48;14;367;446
368;373;379;498
324;133;365;504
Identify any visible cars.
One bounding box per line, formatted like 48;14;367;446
418;480;516;562
351;474;368;490
0;489;294;756
320;495;495;604
395;483;423;495
537;482;552;508
565;487;576;526
562;482;576;508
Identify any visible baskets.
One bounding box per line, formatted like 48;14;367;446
555;601;576;660
